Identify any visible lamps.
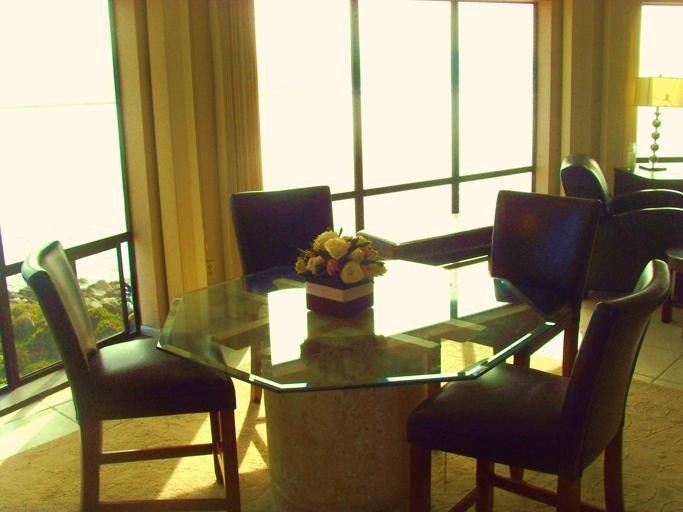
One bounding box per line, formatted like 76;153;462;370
633;76;683;172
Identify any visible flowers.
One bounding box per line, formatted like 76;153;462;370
294;228;385;281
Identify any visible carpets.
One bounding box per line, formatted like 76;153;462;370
0;340;682;512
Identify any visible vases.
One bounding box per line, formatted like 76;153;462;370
306;276;374;317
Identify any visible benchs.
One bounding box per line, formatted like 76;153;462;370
357;212;491;260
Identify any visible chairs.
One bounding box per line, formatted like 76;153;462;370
403;260;670;511
20;240;242;511
561;157;682;295
489;191;602;375
232;184;334;402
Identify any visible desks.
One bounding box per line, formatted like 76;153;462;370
662;248;683;323
613;163;682;195
